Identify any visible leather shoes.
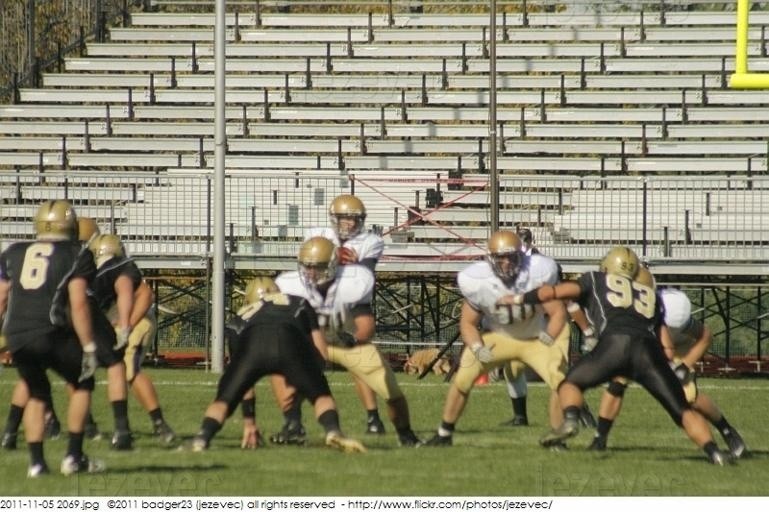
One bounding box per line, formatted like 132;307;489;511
77;342;97;382
327;330;355;347
533;330;554;347
581;338;599;355
471;342;497;363
113;326;131;351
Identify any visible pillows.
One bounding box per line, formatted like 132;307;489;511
34;200;125;270
601;246;691;328
241;237;340;305
329;195;365;240
489;231;531;282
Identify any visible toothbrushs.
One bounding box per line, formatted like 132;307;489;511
335;246;351;266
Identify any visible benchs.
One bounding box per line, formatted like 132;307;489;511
0;1;769;282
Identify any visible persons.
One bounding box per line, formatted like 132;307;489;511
2;194;751;478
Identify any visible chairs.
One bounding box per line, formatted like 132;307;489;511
498;415;528;426
59;455;107;476
112;420;211;452
398;429;452;448
540;402;607;453
711;428;745;466
1;414;96;449
27;463;49;478
326;430;368;454
368;413;384;433
270;420;306;444
